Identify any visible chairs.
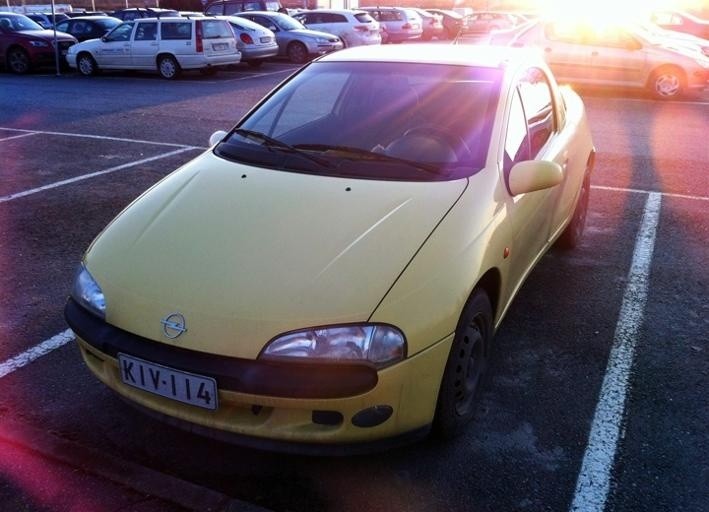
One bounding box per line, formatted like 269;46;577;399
141;27;156;40
333;85;492;161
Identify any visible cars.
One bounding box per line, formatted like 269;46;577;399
63;38;601;450
